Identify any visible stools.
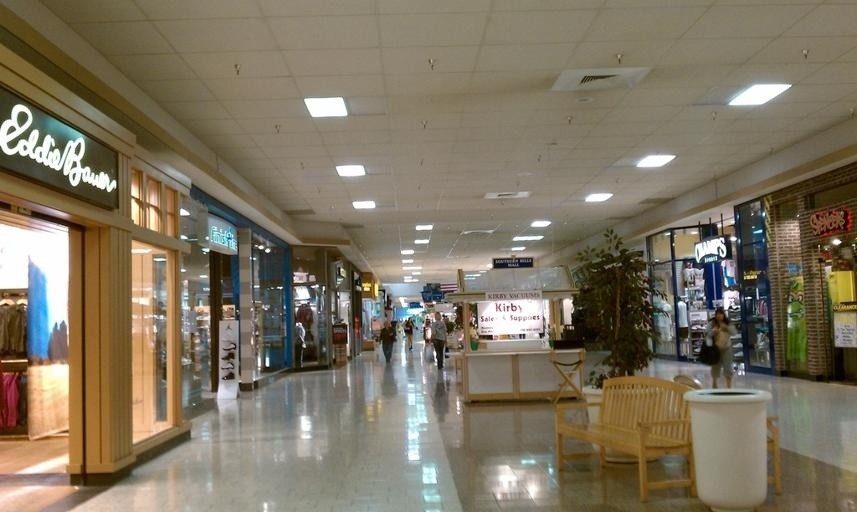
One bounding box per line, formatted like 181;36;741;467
552;359;587;404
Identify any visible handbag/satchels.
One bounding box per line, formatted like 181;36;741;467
700;342;720;365
391;336;396;342
301;342;307;349
433;330;436;336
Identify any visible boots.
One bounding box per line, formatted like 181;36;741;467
222;353;234;360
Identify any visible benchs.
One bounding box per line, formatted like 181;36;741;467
556;377;697;503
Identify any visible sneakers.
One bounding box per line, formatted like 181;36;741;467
730;334;742;339
733;352;743;357
223;373;234;379
223;343;236;350
732;343;743;348
221;363;234;369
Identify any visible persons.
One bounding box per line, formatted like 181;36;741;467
708;306;738;389
429;311;448;370
676;295;690;358
379;319;398;365
402;316;415;350
